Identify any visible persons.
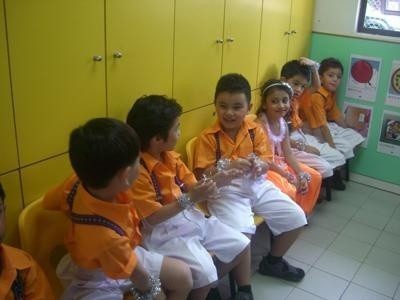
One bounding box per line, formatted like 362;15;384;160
280;56;347;203
193;73;308;300
41;116;193;300
125;95;252;300
0;181;56;300
255;78;322;227
310;57;366;191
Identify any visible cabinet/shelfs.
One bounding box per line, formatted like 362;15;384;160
173;0;264;123
3;1;174;174
0;170;24;253
256;1;316;91
2;1;19;176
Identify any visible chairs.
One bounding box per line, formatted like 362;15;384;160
195;201;212;217
255;215;265;228
20;195;72;296
186;136;199;174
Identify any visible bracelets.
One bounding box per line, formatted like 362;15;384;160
297;172;312;183
242;152;262;183
285;172;296;184
129;272;162;300
296;140;306;151
216;158;231;172
329;143;336;149
177;192;193;211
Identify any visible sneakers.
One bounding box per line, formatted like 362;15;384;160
234;290;253;299
259;256;305;282
